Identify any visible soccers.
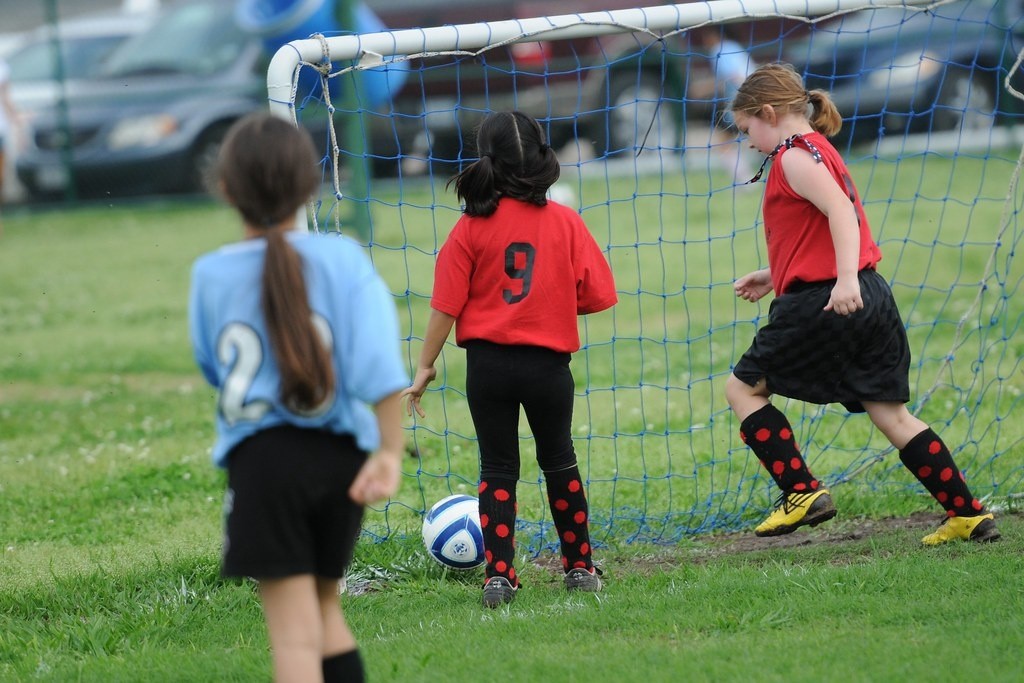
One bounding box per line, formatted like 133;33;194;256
422;494;488;571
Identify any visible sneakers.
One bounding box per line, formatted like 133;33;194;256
483;575;521;609
756;481;837;538
921;508;1001;544
563;568;607;596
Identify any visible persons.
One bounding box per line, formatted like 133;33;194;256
0;61;29;209
704;25;761;191
399;110;618;609
725;64;999;544
191;115;408;682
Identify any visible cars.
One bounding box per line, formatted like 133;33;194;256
784;0;1024;142
0;0;691;202
